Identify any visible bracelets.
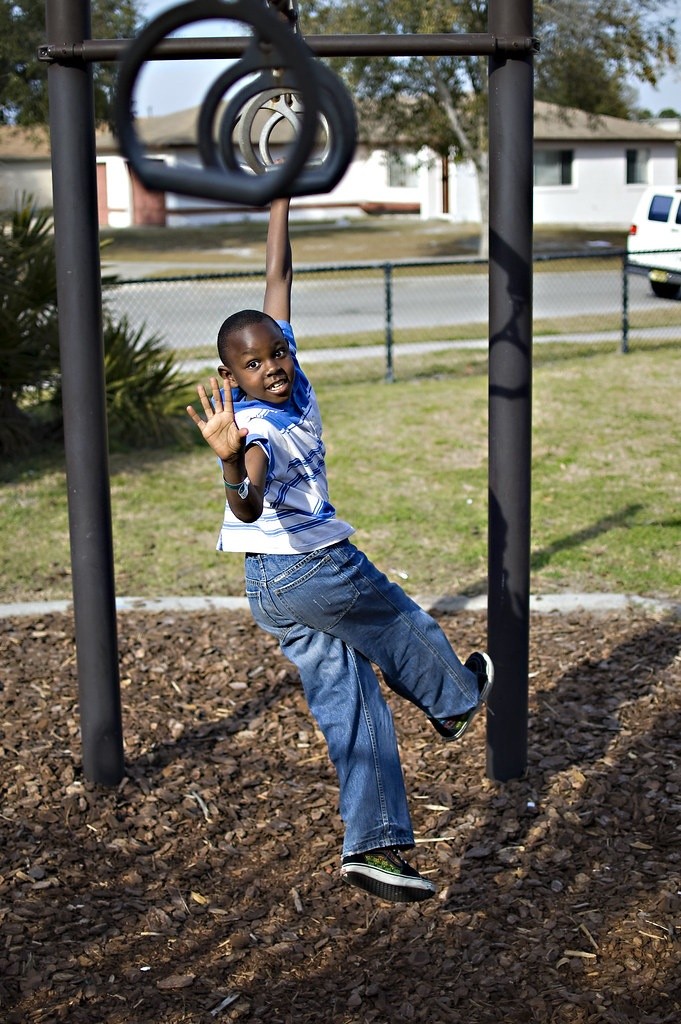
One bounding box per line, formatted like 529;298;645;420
223;469;251;500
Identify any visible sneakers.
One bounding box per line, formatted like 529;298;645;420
431;652;495;740
340;847;437;903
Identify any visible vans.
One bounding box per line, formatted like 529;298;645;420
623;182;681;300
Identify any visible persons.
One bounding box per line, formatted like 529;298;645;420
186;157;494;903
